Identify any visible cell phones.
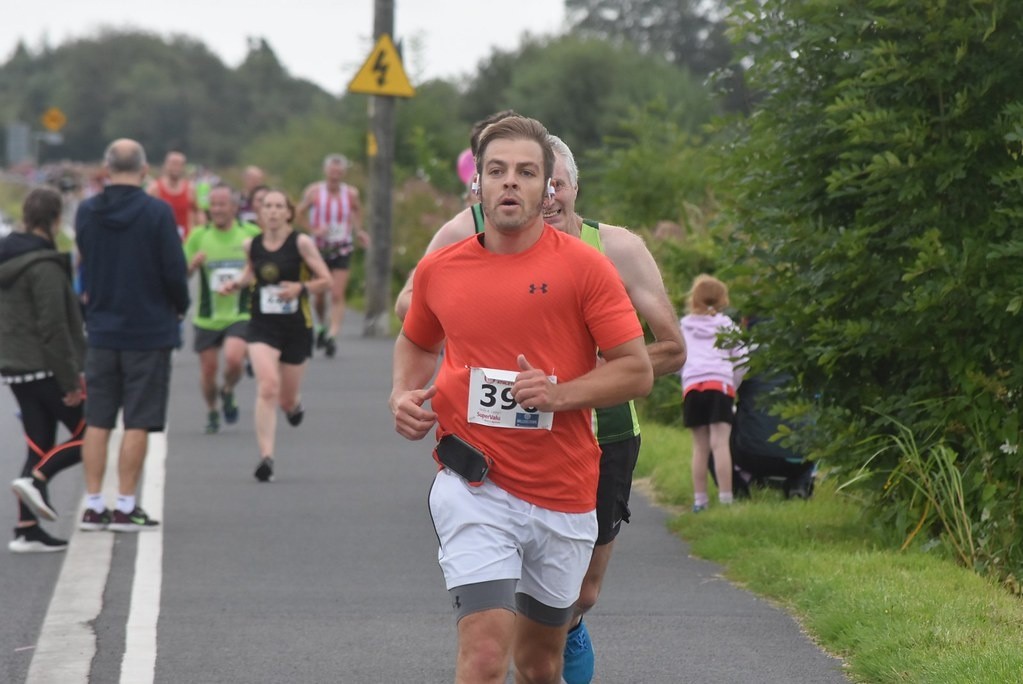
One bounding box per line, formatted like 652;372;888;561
432;432;492;488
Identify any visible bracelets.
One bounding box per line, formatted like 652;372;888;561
301;284;307;295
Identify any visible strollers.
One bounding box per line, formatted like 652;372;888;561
707;368;821;502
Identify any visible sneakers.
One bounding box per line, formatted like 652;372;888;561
559;619;595;684
11;475;58;521
108;506;159;532
80;507;111;530
8;524;68;552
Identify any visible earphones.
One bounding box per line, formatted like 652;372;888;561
546;178;555;199
472;174;480;196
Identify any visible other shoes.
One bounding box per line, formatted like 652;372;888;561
315;324;326;347
246;356;253;376
287;404;305;426
326;337;336;355
255;457;274;481
204;413;220;434
221;388;240;424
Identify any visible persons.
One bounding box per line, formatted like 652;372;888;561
74;138;190;532
677;275;749;513
298;152;371;356
0;189;85;554
219;188;333;482
391;109;685;684
23;149;276;434
707;306;822;498
